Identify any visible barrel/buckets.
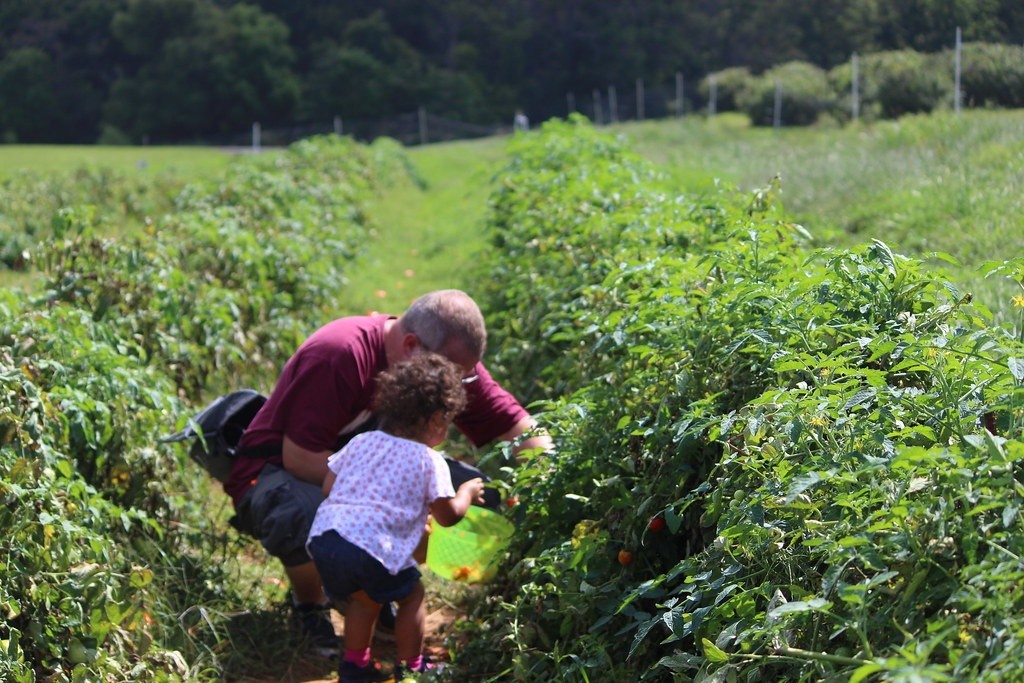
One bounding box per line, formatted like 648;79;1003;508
425;506;516;583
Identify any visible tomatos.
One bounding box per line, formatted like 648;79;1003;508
450;490;746;579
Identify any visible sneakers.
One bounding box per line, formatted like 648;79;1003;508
394;657;446;683
286;587;340;661
375;601;400;643
339;658;394;683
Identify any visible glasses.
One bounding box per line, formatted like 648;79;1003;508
461;365;479;383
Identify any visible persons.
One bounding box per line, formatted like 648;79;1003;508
224;289;558;657
305;354;485;683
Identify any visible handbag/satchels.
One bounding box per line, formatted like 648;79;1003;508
159;389;267;482
